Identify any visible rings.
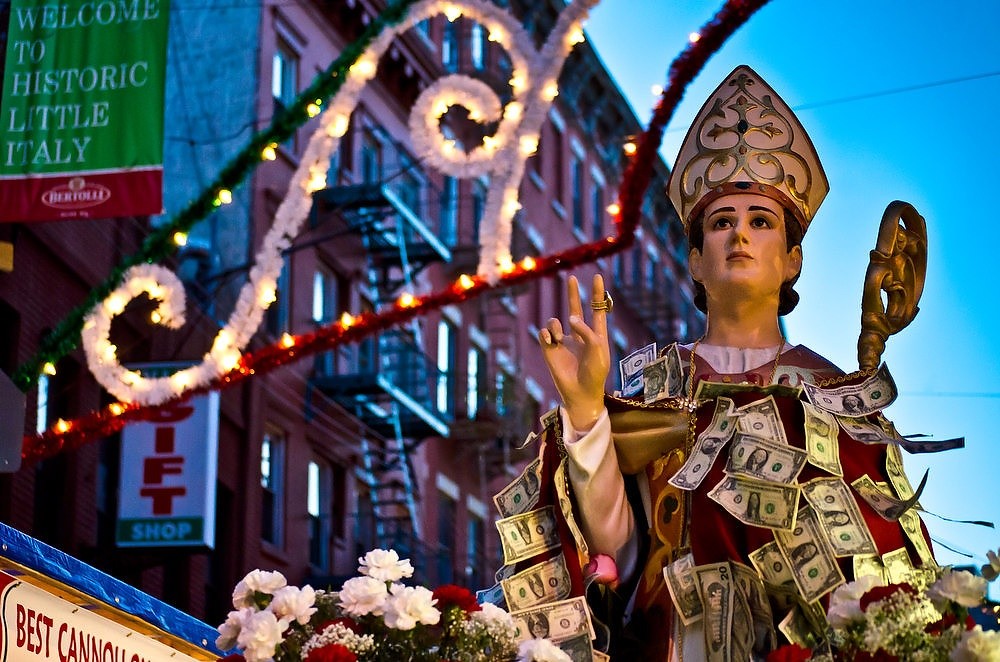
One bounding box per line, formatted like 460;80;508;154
589;290;615;313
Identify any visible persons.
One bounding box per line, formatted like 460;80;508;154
527;175;952;661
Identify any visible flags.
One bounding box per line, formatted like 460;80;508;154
0;0;169;224
